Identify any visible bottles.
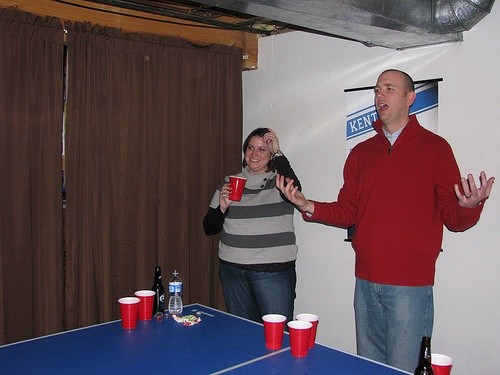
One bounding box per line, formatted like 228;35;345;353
168;269;183;314
414;336;434;375
152;266;165;316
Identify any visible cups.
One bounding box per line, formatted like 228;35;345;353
134;290;156;321
228;175;247;202
431;353;453;375
118;297;140;330
262;314;286;351
287;320;313;359
296;313;318;350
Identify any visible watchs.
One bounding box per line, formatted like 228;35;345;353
273;150;282;157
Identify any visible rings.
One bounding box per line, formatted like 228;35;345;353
465;194;471;198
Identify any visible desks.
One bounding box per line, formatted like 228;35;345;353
0;303;411;375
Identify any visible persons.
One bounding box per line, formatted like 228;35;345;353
276;69;496;374
203;129;302;332
